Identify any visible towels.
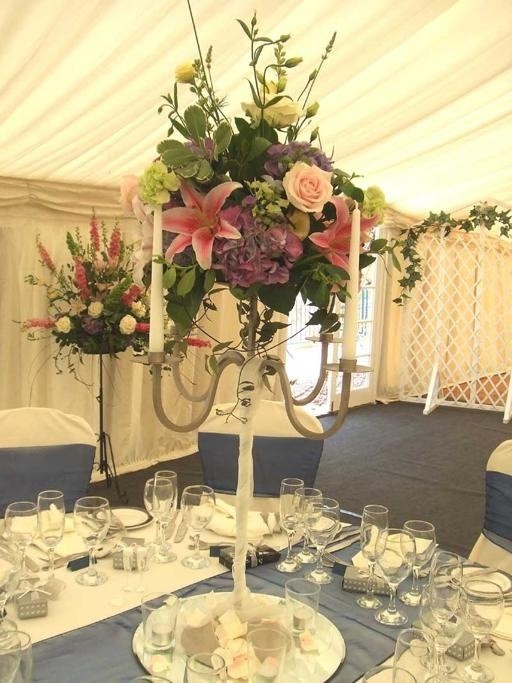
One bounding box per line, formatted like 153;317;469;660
194;495;280;540
352;525;433;569
12;504;92;557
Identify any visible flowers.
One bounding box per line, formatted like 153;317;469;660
11;208;209;387
132;0;512;358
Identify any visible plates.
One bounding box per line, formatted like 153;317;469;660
445;562;512;600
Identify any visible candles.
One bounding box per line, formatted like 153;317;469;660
276;310;290;360
341;196;360;361
146;198;169;353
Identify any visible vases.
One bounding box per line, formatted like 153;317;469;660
77;332;126;355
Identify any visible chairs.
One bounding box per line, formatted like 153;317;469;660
1;406;96;518
461;438;512;570
199;402;326;504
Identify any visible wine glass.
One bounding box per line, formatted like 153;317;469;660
1;470;501;683
